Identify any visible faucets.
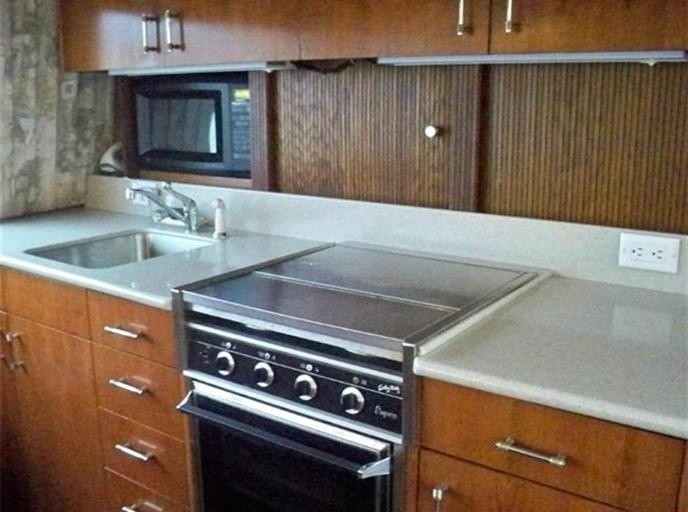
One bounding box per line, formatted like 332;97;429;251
125;182;198;231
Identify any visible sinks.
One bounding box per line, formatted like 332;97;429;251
24;228;217;269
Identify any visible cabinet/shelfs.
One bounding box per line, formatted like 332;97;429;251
296;2;687;63
56;0;299;73
0;266;203;512
405;377;688;512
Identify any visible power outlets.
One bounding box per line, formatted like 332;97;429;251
617;233;682;275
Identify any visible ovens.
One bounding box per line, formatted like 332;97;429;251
182;322;402;512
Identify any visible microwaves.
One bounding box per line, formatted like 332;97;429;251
125;78;254;181
